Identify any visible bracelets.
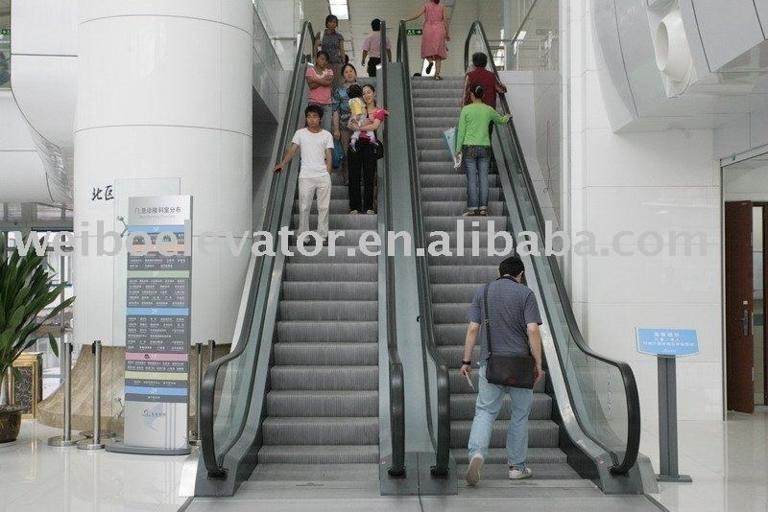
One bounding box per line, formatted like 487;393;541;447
462;360;471;365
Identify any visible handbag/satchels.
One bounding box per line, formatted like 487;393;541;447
486;354;536;389
334;138;344;169
355;140;384;161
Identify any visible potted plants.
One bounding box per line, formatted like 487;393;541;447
0;245;76;443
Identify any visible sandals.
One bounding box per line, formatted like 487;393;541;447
434;76;443;81
426;62;433;74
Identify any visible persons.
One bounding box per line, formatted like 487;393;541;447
348;85;379;153
464;52;507;173
348;85;384;215
361;19;392;77
313;15;346;82
332;64;390;185
306;51;334;135
461;257;543;486
273;105;334;246
402;0;450;80
456;85;513;216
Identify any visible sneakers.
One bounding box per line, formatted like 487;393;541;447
367;209;374;215
466;454;484;486
509;466;533;479
480;210;489;215
462;209;479;216
350;210;359;214
